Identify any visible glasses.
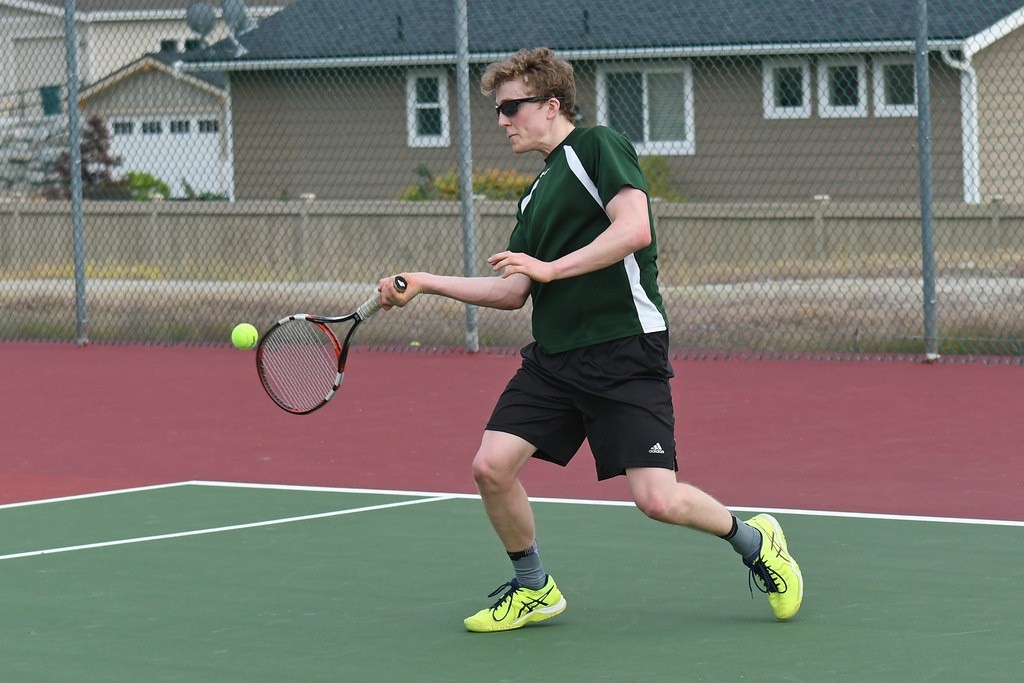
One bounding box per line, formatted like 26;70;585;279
494;96;566;117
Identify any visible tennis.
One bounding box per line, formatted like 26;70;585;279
230;323;260;351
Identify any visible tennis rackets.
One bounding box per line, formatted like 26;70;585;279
256;275;408;416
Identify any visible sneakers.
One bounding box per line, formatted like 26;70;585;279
742;513;804;620
463;573;567;632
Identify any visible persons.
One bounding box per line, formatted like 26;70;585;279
376;48;803;634
